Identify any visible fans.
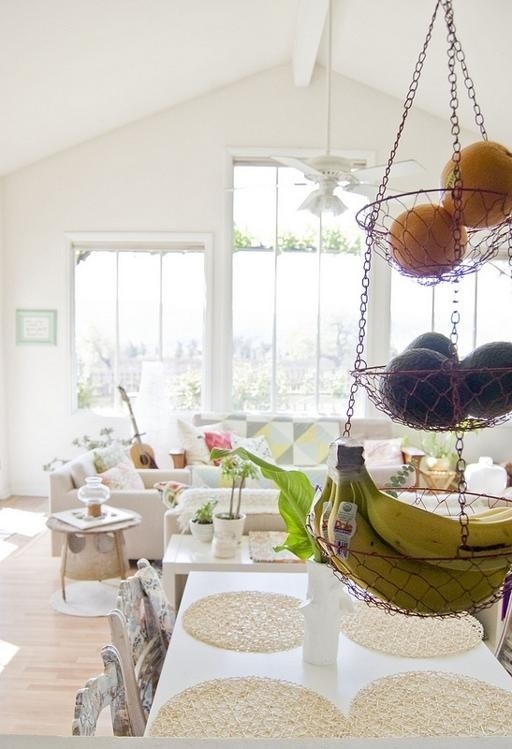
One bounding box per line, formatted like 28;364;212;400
224;0;426;219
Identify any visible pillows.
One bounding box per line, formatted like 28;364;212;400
94;443;125;474
204;430;235;468
231;434;277;469
171;417;228;467
99;459;147;491
360;436;405;466
67;448;98;488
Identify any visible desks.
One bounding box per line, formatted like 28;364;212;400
144;571;512;737
162;533;314;615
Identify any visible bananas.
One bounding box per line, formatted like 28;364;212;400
304;437;512;617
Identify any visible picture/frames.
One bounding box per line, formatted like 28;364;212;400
12;308;60;347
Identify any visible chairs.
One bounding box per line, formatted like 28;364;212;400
133;557;172;651
72;644;134;736
107;556;176;736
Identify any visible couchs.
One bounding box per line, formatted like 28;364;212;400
49;444;192;569
168;412;426;490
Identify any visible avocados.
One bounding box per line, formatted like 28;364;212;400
380;332;512;428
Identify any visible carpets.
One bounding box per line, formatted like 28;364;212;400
49;578;122;617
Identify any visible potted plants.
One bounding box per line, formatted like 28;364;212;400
191;497;219;543
212;457;262;560
421;418;481;471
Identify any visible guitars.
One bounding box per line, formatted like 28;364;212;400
117;385;158;469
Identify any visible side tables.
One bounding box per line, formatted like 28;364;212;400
47;503;143;602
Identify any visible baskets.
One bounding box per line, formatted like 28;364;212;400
349;365;511;431
355;188;511;278
305;488;512;620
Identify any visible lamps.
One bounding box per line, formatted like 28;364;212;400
296;153;355;218
